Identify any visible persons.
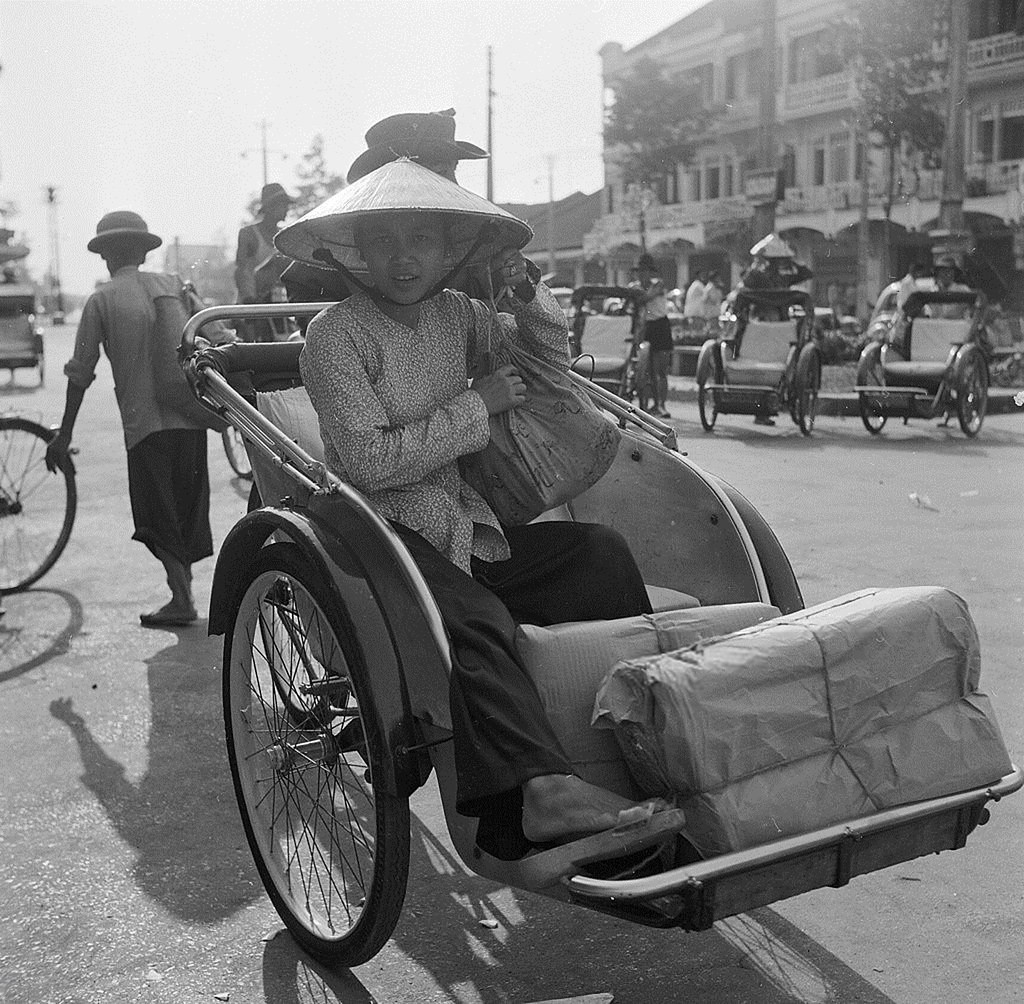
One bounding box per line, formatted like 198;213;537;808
628;253;673;418
924;255;971;320
281;109;491;331
898;262;926;309
273;156;653;844
44;210;241;624
742;233;812;322
234;182;297;304
685;270;725;319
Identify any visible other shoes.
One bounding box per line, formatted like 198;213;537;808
754;416;774;425
658;406;670;418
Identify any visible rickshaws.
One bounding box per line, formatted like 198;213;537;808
566;284;652;414
176;302;1024;970
853;289;993;439
695;290;823;436
0;285;45;389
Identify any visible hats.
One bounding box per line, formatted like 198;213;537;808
750;234;796;258
88;211;161;254
631;254;658;272
272;157;534;278
258;184;296;214
346;108;489;183
930;256;963;276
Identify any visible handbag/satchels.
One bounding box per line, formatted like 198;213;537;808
156;295;233;432
460;340;621;525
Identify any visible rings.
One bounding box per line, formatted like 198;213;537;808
511;266;516;276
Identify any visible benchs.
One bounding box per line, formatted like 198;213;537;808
0;316;39;367
673;345;702;375
572;315;635;383
261;382;772;888
705;320;804;392
855;316;975;392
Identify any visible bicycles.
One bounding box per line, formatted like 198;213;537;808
0;420;77;598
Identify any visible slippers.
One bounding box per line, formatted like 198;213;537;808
519;794;685;891
140;610;196;627
